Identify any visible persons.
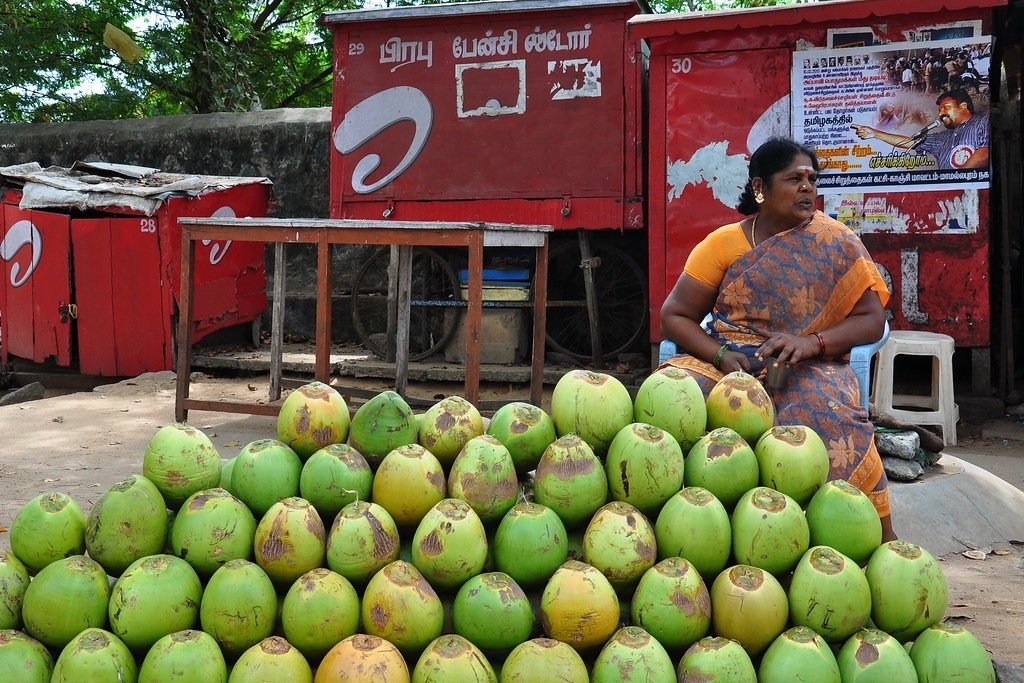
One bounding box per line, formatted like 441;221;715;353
851;43;990;170
661;137;899;545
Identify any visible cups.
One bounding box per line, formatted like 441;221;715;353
767;355;792;388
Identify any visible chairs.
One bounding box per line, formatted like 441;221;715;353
658;313;890;419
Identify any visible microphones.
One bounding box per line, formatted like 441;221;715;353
911;120;942;139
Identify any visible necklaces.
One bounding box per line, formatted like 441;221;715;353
751;216;758;248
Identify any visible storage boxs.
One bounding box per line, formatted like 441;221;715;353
458;261;530;302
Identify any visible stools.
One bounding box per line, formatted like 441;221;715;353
867;330;960;448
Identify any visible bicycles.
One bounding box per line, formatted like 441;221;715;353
349;241;650;364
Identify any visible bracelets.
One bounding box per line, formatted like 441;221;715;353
808;332;825;359
714;344;731;370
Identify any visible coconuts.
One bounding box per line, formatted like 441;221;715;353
1;366;996;682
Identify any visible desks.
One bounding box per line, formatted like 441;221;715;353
174;216;554;426
